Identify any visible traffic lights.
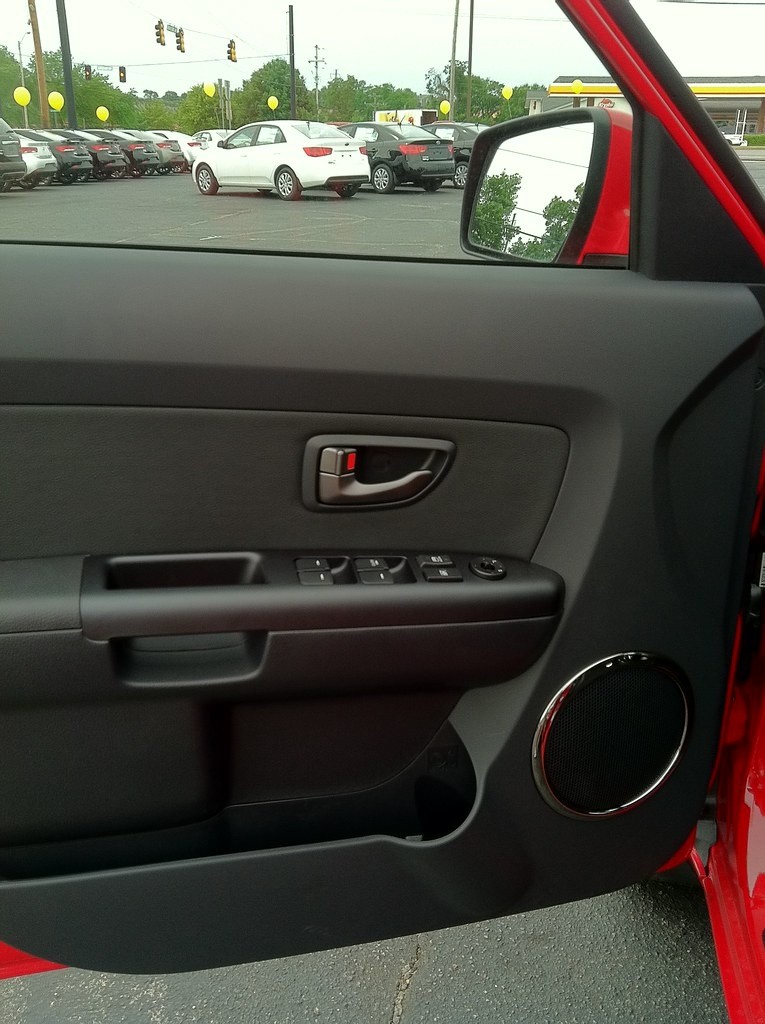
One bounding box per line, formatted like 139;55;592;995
175;27;184;52
85;65;92;81
155;19;166;46
227;39;237;62
119;66;126;83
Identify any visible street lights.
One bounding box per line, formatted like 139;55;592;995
17;31;32;127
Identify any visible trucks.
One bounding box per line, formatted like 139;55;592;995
374;108;458;143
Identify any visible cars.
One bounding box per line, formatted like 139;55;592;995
336;120;456;194
191;118;371;201
421;120;491;190
0;117;253;196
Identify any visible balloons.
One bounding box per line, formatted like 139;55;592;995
96;105;109;121
572;80;583;94
267;96;278;111
48;91;65;110
502;87;513;99
13;87;30;107
440;100;450;115
204;80;215;97
